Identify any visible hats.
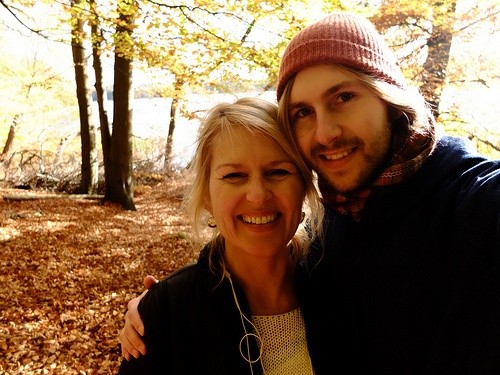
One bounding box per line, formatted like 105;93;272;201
277;12;407;105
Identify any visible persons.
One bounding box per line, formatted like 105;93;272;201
118;97;401;374
119;12;500;375
